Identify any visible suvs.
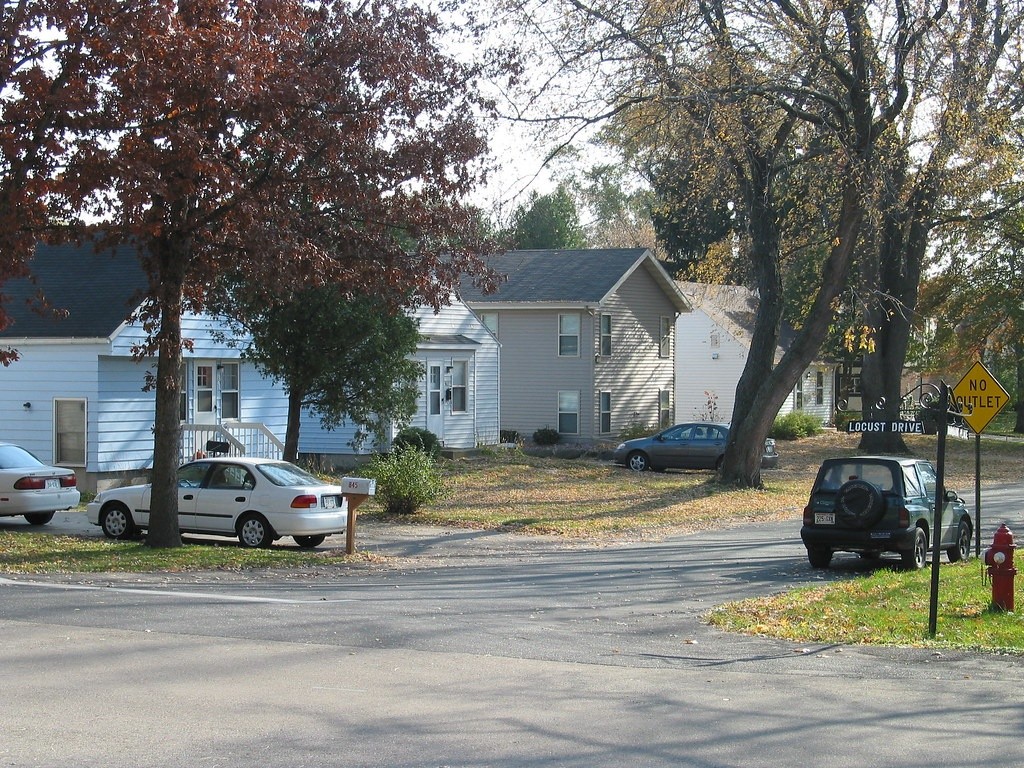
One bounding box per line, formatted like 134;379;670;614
800;454;975;572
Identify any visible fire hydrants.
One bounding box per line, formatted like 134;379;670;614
980;522;1017;615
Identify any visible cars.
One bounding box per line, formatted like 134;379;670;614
0;441;81;526
613;421;780;472
88;457;356;550
